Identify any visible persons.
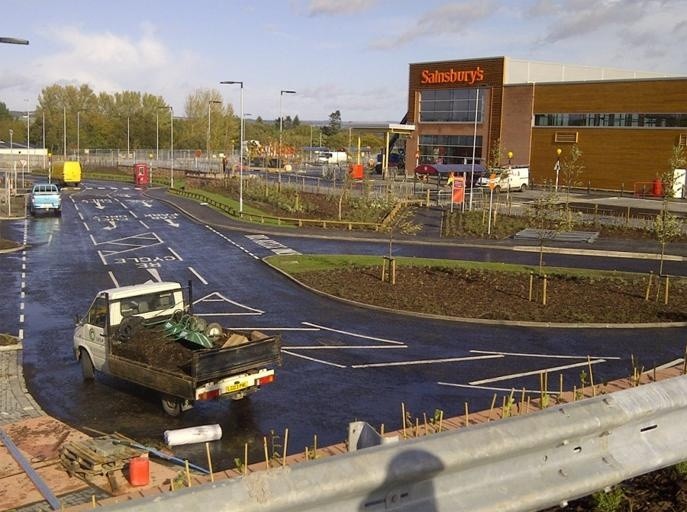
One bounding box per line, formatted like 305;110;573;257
221;155;228;171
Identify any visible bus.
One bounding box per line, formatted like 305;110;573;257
51;160;81;185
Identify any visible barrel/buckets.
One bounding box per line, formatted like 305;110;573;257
129;457;150;486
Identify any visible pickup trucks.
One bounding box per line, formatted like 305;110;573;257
73;282;281;417
30;184;62;216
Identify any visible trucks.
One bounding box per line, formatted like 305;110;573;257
316;151;347;165
476;165;529;193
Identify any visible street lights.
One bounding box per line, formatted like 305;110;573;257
221;81;243;218
76;108;86;161
555;148;562;192
208;100;220;152
149;153;153;186
278;91;296;193
155;106;174;187
506;152;513;204
48;153;51;182
